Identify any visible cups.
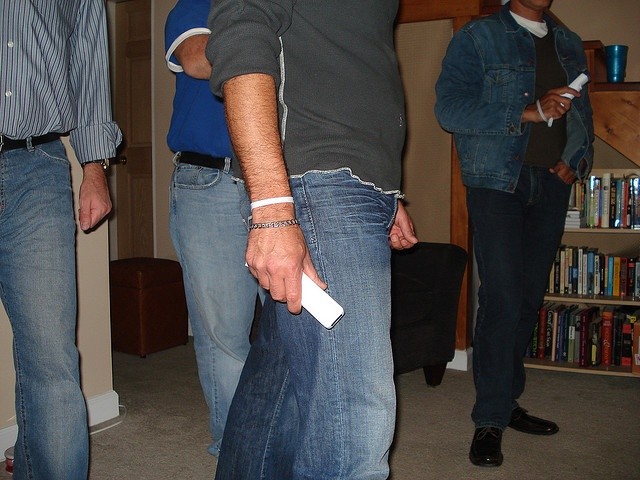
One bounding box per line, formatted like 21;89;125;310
606;44;627;82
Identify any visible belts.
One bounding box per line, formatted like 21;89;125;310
179;151;232;171
0;131;62;152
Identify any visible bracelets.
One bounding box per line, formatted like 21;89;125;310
250;218;297;231
249;196;294;209
536;99;548;123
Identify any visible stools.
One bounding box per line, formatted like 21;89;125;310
110;257;188;358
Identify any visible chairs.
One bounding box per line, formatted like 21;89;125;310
391;242;467;385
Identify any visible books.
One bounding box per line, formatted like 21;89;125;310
526;170;640;371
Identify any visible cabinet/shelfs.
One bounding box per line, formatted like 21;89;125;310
523;168;640;378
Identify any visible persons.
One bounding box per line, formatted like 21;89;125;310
3;1;125;475
436;1;593;470
203;0;421;480
164;1;271;458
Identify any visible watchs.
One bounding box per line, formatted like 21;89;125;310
81;157;110;170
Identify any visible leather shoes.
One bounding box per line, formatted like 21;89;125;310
469;427;503;467
507;407;559;435
